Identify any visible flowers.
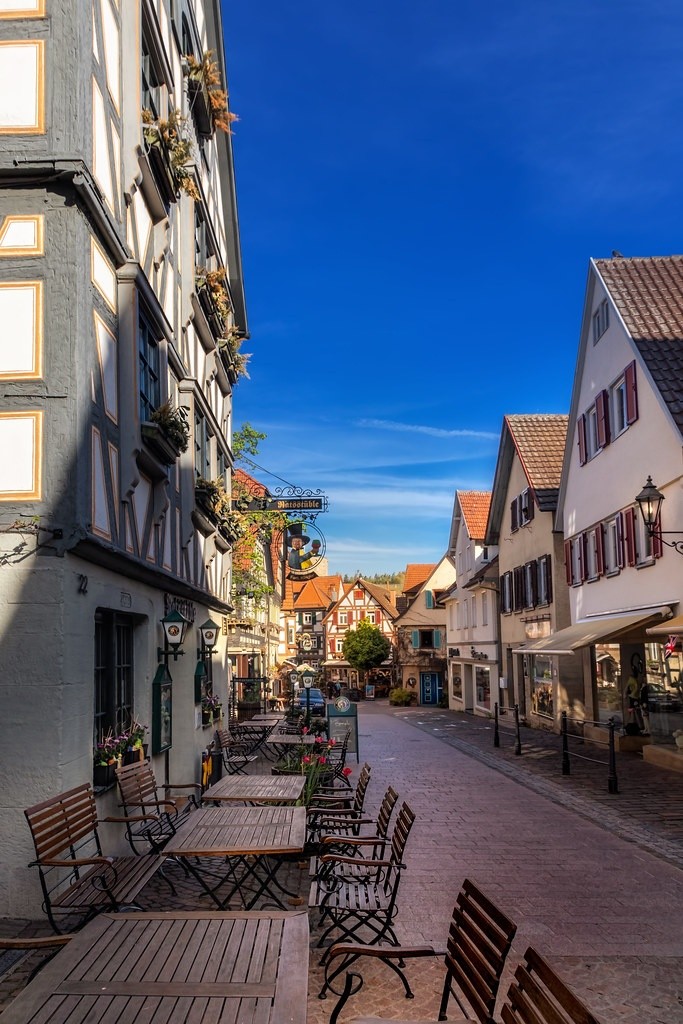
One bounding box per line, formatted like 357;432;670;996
263;753;352;806
137;722;149;744
92;726;111;766
126;713;140;752
200;694;222;713
105;722;123;764
118;729;133;752
284;726;336;771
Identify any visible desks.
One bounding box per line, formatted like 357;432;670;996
238;720;279;763
202;775;307;807
252;715;285;755
162;806;307;911
266;735;316;763
0;911;311;1024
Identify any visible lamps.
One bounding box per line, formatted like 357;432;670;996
635;474;683;556
157;608;190;662
197;618;222;660
247;591;254;598
236;587;246;595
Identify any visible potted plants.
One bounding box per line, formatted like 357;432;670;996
195;473;250;542
143;393;192;465
141;107;204;204
268;696;277;712
185;48;241;142
195;267;254;384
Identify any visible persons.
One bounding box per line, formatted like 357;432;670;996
326;678;341;700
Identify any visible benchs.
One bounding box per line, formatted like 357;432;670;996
22;713;600;1024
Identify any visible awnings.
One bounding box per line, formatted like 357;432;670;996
646;614;683;634
512;607;673;655
284;661;297;672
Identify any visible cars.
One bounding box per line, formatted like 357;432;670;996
647;682;681;713
298;688;326;717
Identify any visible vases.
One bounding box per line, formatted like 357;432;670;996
92;764;107;786
204;710;212;722
212;707;220;718
270;764;333;787
106;761;117;785
202;712;209;724
121;751;133;766
141;743;148;758
217;706;221;715
131;749;140;762
